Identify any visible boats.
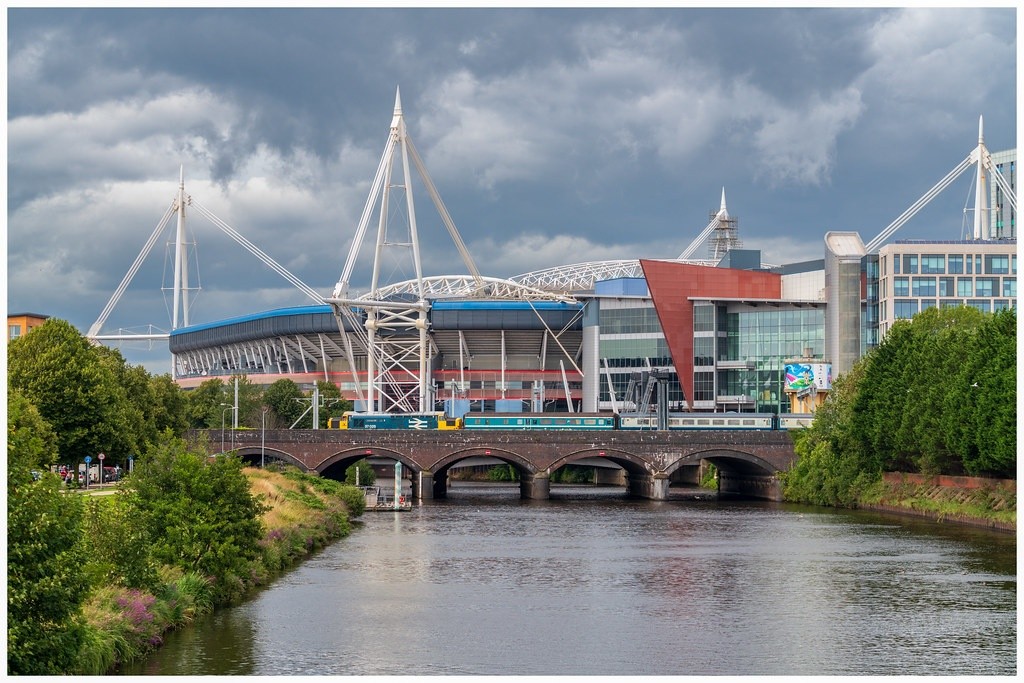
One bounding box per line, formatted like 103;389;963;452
356;461;413;512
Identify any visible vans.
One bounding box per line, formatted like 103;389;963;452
79;464;100;481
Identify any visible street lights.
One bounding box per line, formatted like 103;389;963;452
222;406;240;455
221;402;234;453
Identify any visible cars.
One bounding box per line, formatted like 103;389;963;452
65;470;91;485
54;465;69;479
104;466;117;482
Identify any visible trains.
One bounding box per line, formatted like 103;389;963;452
326;411;816;430
